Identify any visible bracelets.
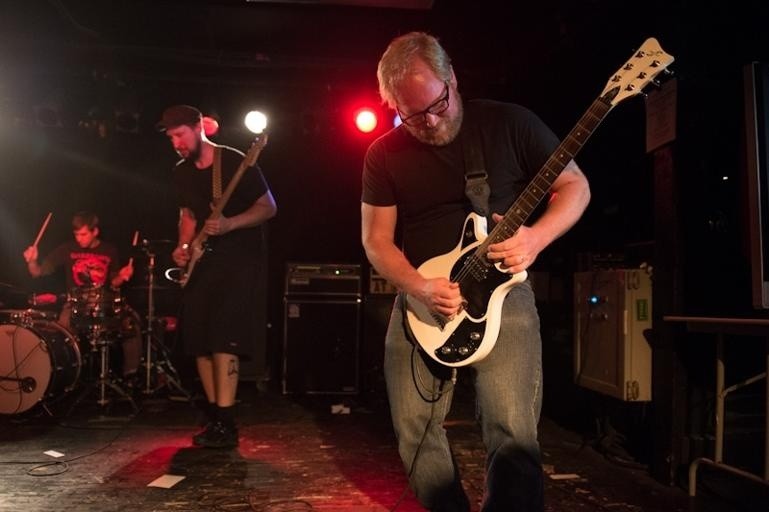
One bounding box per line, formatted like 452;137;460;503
177;241;192;250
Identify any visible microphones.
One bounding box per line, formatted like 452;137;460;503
127;231;139;257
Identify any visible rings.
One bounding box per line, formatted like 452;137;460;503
519;254;524;262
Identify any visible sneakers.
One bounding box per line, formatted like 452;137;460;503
193;423;215;443
202;427;239;447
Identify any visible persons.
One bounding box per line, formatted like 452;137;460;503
23;209;159;397
360;29;592;512
154;104;278;451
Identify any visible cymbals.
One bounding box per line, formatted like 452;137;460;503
141;239;174;243
1;283;35;295
3;308;57;318
131;285;170;289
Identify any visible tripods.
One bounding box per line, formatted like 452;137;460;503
59;333;143;428
131;267;196;408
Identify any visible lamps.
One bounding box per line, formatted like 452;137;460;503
2;41;229;141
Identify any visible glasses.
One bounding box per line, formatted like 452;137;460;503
395;83;450;125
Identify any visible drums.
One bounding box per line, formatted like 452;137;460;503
0;319;83;422
68;289;127;326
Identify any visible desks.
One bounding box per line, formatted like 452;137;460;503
663;312;769;496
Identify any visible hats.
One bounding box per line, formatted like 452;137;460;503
156;105;201;132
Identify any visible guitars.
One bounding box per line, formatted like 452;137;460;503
401;37;675;368
178;129;270;288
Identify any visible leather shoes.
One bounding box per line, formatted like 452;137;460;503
122;376;140;389
